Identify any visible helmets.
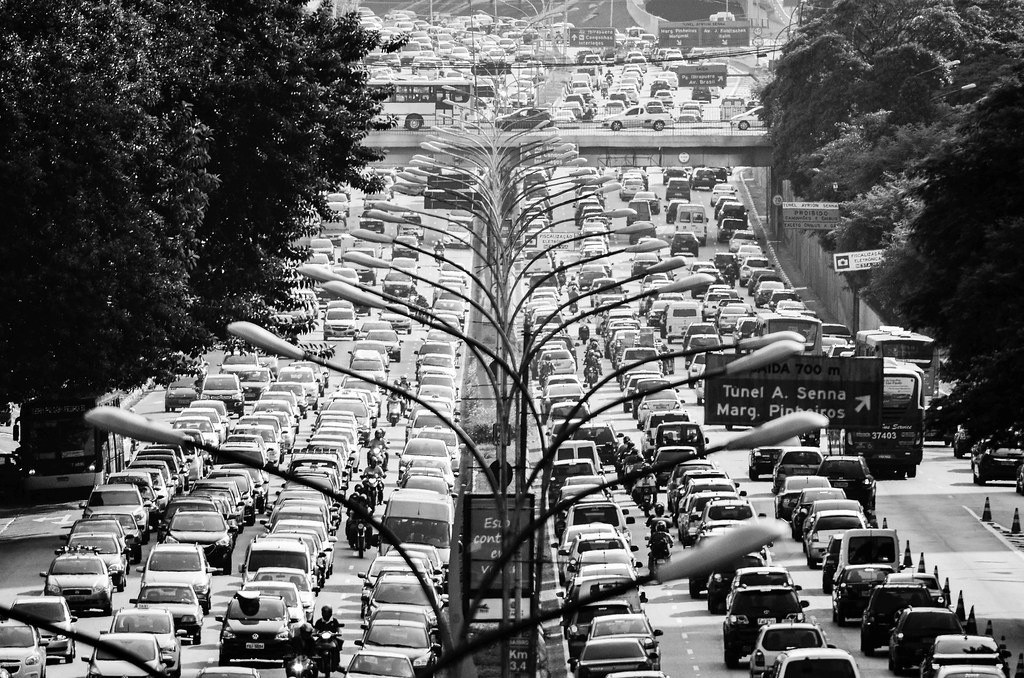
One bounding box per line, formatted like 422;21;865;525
655;520;667;532
370;456;377;462
351;484;368;500
624;436;630;442
628;441;635;446
572;285;577;290
655;503;664;514
589;349;594;356
438;239;441;242
321;605;332;617
637;453;644;460
581;310;585;313
393;379;400;385
400;375;408;380
591;341;598;348
632;447;638;452
560;260;565;264
375;428;386;436
571;276;575;280
641;462;651;469
299;623;313;636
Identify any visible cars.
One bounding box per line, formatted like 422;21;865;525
730;105;774;130
512;167;1023;678
494;105;554;132
0;168;475;677
356;7;709;124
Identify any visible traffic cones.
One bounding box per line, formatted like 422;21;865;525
986;620;994;636
883;518;887;528
864;505;878;529
1011;508;1021;534
917;552;926;573
966;605;977;635
955;591;965;621
934;564;939;580
903;540;913;568
997;634;1008;667
981;497;993;521
1015;652;1024;678
942;577;952;605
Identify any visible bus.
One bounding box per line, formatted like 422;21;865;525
347;74;473;131
13;388;137;505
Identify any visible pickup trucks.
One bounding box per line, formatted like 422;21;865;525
609;102;675;131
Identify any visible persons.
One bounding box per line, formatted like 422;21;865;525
601;69;614;95
415;294;430;323
281;605;342;678
434;239;445;260
553;260;604;378
614;435;675;575
345;373;413;539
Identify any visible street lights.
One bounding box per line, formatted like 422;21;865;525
85;1;830;676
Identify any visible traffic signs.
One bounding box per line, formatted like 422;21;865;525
521;142;579;165
676;64;727;87
569;26;616;47
704;350;884;433
660;21;750;47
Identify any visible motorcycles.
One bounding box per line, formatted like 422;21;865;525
613;446;675;586
582;349;604;389
558;273;581;315
281;624;345;677
349;442;391;558
576;321;591;345
386;382;411;427
434;248;445;267
